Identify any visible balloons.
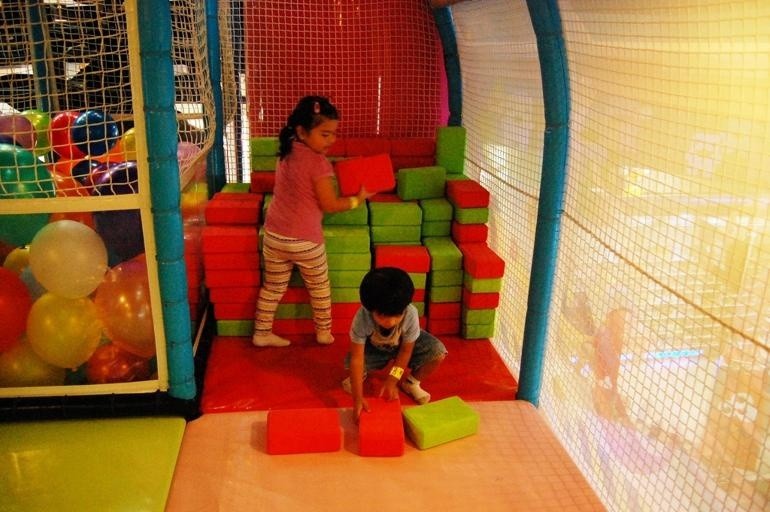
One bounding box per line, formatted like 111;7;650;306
1;109;208;387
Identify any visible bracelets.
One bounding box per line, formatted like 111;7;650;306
350;196;359;210
388;366;405;381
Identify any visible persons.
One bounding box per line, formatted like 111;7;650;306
339;265;448;428
247;94;378;349
